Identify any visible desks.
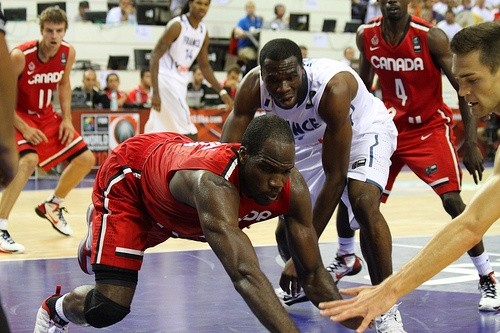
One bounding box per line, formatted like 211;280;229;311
1;0;361;175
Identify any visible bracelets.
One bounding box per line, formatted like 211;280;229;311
219;89;228;96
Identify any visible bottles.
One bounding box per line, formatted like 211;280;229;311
110;90;118;111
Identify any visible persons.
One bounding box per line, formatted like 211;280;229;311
325;0;500;315
0;0;187;254
33;114;374;333
217;38;406;333
319;22;500;333
183;0;362;110
144;0;234;144
353;0;500;45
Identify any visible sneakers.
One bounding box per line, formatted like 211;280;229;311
35;200;72;236
375;301;407;333
77;203;95;275
325;253;362;284
277;288;309;306
478;271;500;311
0;229;24;254
33;285;70;333
